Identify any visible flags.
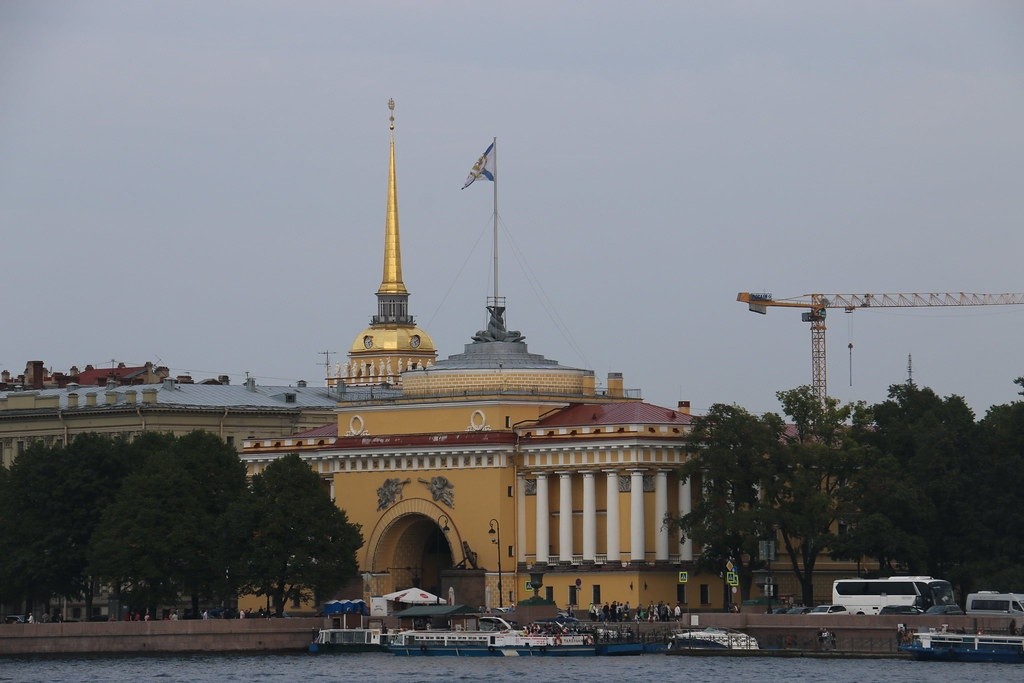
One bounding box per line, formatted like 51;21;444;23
461;142;494;191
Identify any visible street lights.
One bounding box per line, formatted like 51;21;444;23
436;515;451;606
487;517;502;608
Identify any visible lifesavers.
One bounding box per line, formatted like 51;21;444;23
421;645;427;653
488;646;495;652
540;647;546;653
554;638;562;644
586;636;593;645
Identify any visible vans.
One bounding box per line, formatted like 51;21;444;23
965;590;1024;616
6;615;26;622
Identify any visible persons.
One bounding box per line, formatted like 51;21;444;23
896;627;913;652
25;605;267;623
817;627;837;651
382;600;682;645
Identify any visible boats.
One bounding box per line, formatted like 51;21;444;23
896;622;1024;663
669;627;760;650
313;611;671;656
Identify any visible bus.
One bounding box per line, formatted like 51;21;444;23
831;577;956;615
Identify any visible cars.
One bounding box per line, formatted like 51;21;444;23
91;614;108;622
211;608;239;619
786;607;814;614
807;604;851;615
879;605;926;616
919;605;965;615
764;608;788;614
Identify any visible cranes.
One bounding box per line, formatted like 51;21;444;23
734;290;1024;425
